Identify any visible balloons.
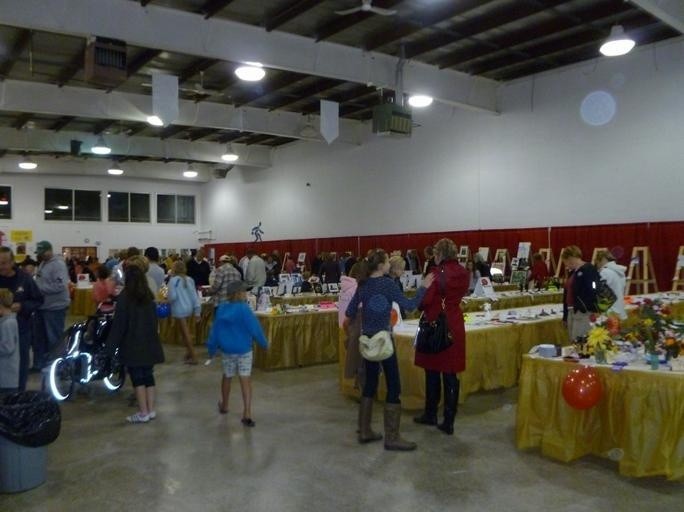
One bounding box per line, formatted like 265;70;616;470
561;364;604;409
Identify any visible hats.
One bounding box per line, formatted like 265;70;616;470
219;254;231;261
227;280;254;295
34;241;52;253
122;255;149;274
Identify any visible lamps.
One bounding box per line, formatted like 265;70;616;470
180;165;199;178
90;134;112;156
106;159;124;176
16;155;41;172
220;142;239;163
596;23;637;59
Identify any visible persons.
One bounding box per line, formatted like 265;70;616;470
207;283;268;426
103;263;165;424
345;249;436;454
413;239;470;436
1;241;71;390
532;245;628;343
466;252;492;283
70;246;438;365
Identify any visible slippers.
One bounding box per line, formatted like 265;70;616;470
242;417;255;427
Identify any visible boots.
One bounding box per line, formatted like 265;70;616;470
359;397;383;443
384;402;417;451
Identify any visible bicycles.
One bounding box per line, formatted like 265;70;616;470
44;317;125;406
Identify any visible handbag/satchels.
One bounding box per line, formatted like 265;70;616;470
414;312;454;353
359;330;394;362
153;336;164;364
157;304;170;318
201;296;215;321
593;279;616;313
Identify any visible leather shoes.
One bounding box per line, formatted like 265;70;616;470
439;423;453;434
414;415;437;425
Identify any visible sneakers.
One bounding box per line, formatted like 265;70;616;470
148;411;156;420
126;412;150;423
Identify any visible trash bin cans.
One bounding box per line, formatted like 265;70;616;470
0;390;54;494
